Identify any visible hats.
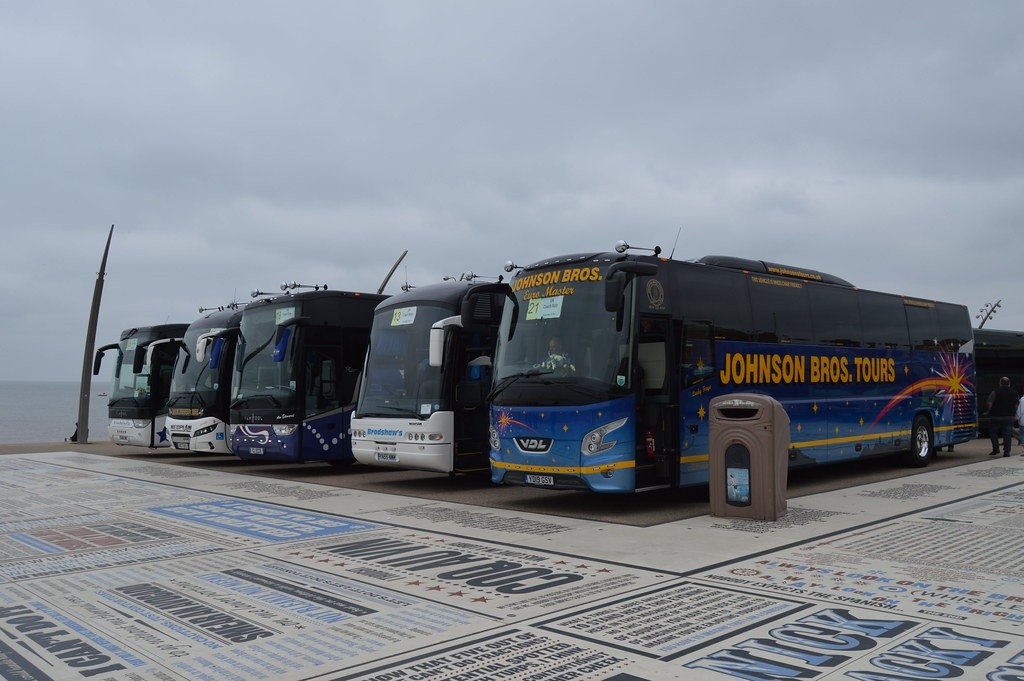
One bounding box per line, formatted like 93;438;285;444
423;358;436;374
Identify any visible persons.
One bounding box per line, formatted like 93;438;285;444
535;337;578;375
936;376;1024;457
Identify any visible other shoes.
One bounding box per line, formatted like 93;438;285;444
989;449;1000;455
1003;451;1009;456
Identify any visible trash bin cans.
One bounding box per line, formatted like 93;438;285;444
709;393;791;521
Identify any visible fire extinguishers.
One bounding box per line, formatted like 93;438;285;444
646;430;655;457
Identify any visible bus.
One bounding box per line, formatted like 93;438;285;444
345;273;510;478
93;323;191;451
972;327;1024;438
228;280;394;467
484;239;978;498
146;301;249;456
